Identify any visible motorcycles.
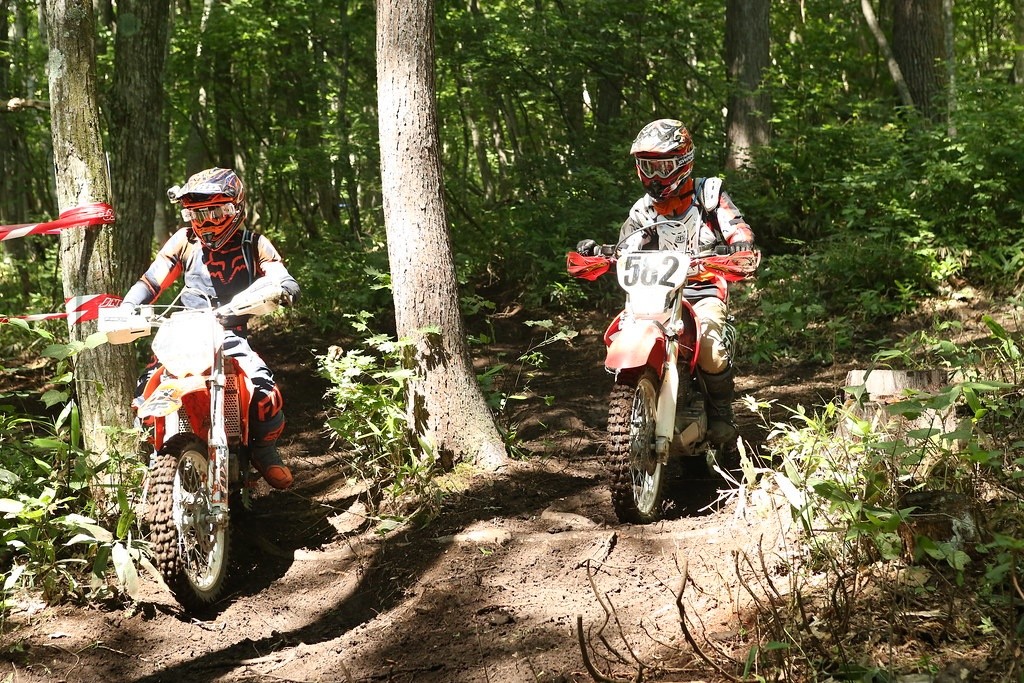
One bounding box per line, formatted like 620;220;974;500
97;302;291;612
564;239;763;526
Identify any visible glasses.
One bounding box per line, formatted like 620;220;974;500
188;204;227;227
638;158;678;179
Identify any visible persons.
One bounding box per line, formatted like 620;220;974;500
575;118;762;442
119;167;300;490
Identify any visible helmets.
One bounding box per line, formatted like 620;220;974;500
629;118;695;203
180;167;246;252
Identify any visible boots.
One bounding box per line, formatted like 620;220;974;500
249;408;293;488
698;359;735;444
